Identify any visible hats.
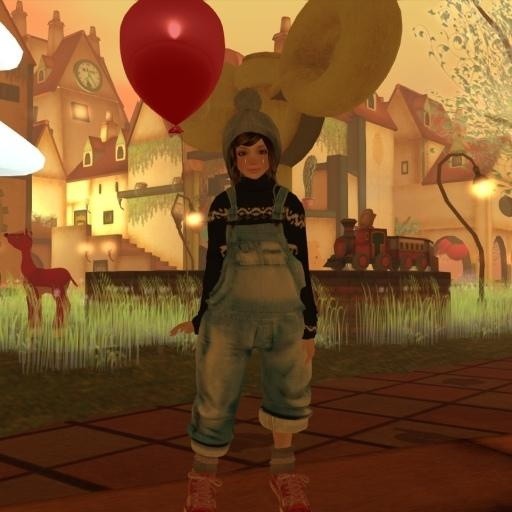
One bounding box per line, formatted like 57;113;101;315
223;88;280;161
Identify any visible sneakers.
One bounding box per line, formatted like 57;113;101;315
184;472;223;512
269;472;313;512
